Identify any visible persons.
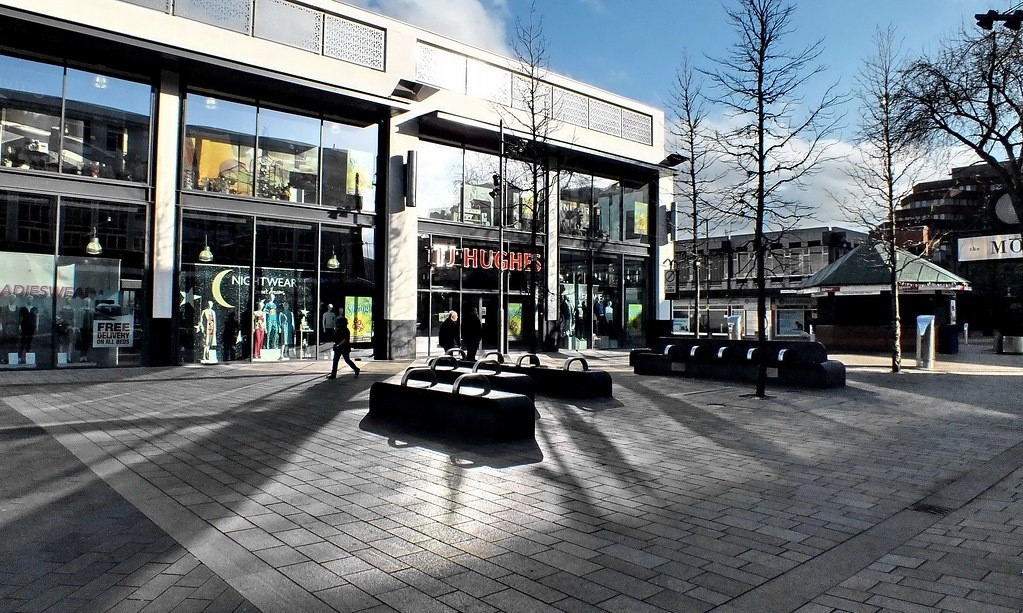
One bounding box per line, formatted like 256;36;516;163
438;310;460;360
321;304;344;341
199;300;218;362
251;292;296;362
0;286;111;364
459;305;483;363
558;291;620;342
324;317;360;379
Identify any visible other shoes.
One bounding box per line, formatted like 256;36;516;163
354;368;360;378
329;370;337;378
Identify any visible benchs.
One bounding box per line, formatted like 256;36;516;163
629;336;846;388
405;354;535;402
429;347;613;399
368;367;535;447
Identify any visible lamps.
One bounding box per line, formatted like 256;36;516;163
328;245;340;269
198;234;213;262
85;226;103;255
